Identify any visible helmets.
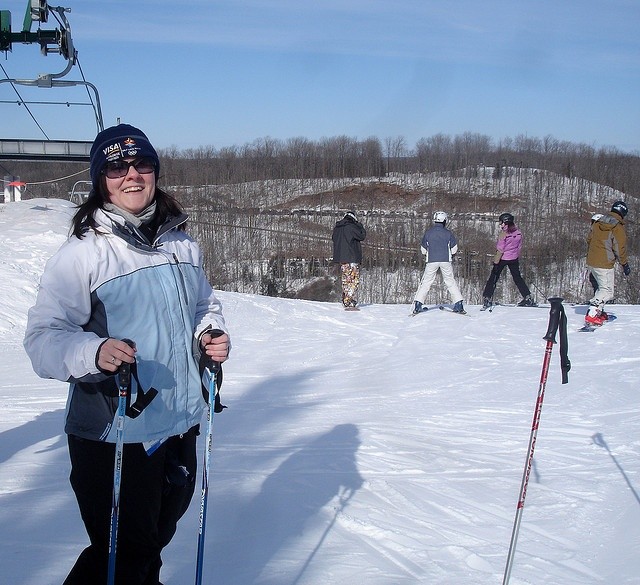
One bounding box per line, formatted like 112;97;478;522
590;213;604;221
344;211;356;220
433;211;448;224
611;201;628;218
499;213;514;224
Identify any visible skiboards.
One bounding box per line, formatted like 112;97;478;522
481;303;554;315
405;303;471;316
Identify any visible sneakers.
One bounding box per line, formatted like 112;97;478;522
414;301;421;310
453;301;463;311
596;308;608;319
519;297;534;306
485;297;492;307
585;305;602;325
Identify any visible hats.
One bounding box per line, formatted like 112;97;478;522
89;123;159;187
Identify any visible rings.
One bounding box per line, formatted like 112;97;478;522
112;357;115;365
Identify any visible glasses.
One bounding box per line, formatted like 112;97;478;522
98;157;157;178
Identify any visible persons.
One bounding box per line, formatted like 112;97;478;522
584;201;631;325
413;211;467;315
332;211;366;308
482;213;533;306
587;213;605;296
22;123;233;585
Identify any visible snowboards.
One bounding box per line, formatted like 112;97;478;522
341;306;359;312
576;315;615;331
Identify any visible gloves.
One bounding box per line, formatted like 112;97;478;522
623;263;630;276
